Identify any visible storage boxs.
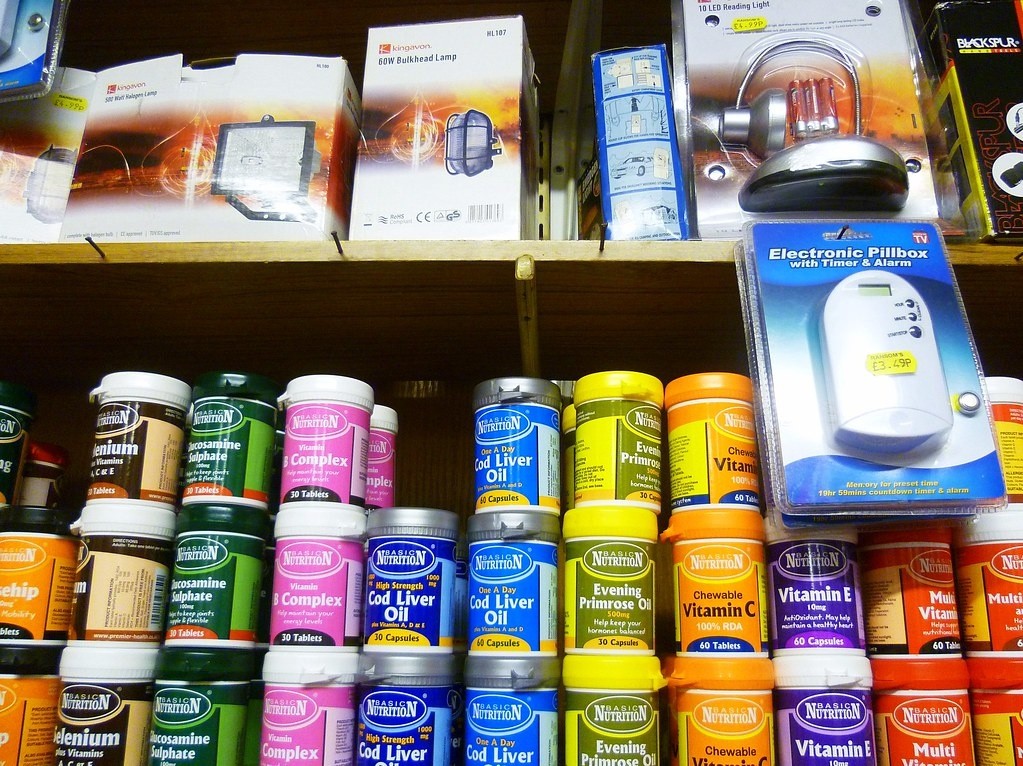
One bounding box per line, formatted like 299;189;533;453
916;0;1023;245
59;52;362;241
682;0;937;242
588;44;690;240
348;16;535;241
0;68;97;245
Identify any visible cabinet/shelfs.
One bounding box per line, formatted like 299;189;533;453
0;0;1023;766
